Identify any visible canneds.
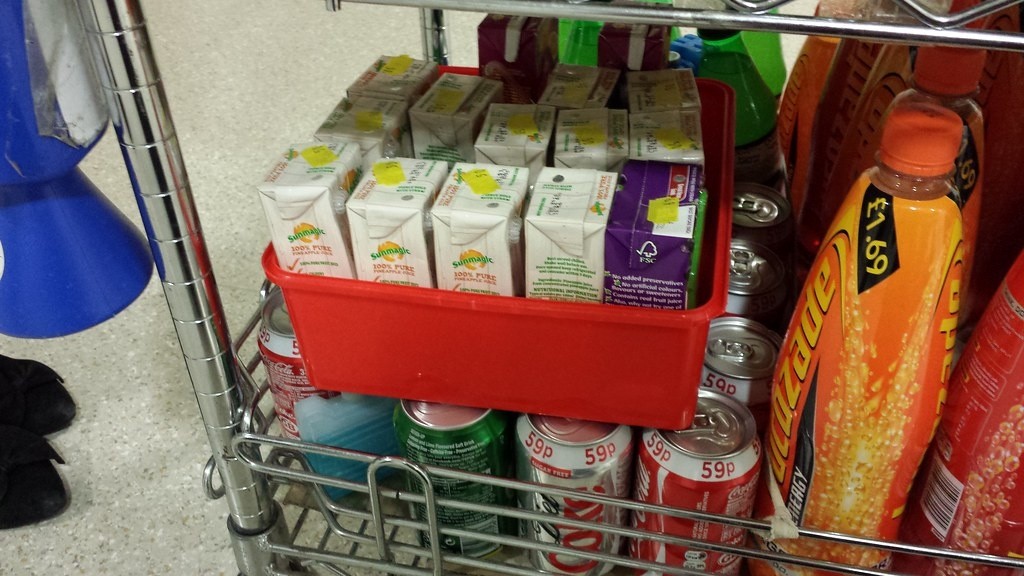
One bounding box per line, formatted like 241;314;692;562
259;181;792;576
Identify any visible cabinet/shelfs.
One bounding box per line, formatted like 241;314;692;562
69;0;1024;576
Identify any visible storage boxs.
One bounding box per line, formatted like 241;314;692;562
262;65;735;429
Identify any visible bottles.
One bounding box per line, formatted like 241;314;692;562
547;1;1024;576
